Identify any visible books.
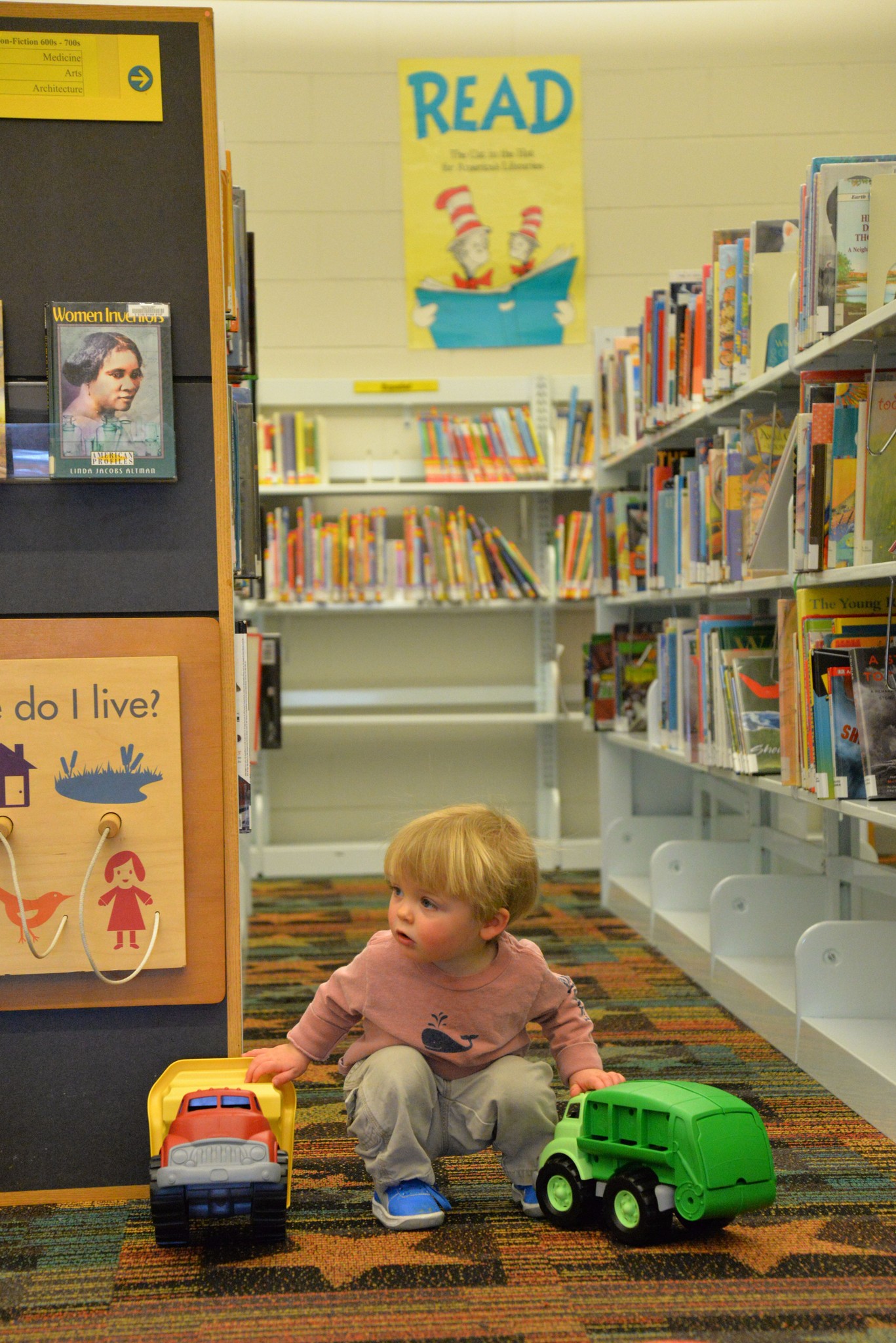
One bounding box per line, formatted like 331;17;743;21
254;406;541;598
545;151;896;808
215;154;276;826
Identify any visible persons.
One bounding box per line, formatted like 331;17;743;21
241;802;626;1228
59;329;157;459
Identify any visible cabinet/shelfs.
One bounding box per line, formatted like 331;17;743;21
583;299;896;1139
256;384;602;877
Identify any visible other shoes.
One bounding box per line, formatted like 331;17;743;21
511;1183;544;1217
372;1178;444;1230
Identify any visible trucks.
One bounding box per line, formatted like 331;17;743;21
531;1076;777;1241
141;1056;297;1247
